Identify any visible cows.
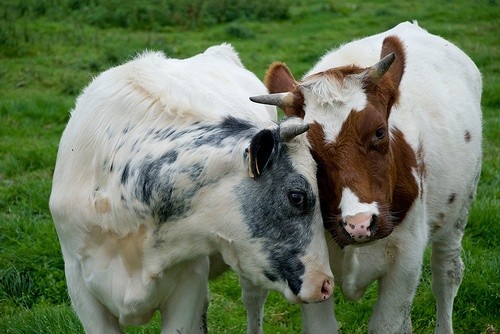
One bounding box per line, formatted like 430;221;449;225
247;18;482;334
48;42;334;334
